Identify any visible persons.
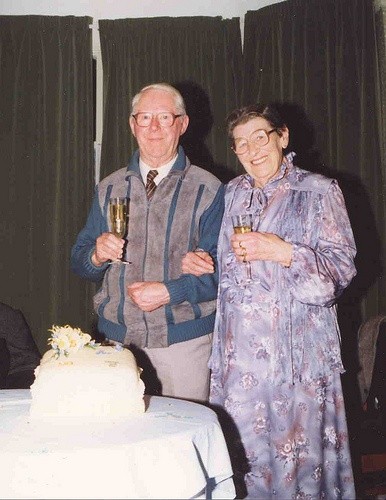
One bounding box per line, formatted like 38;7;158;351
70;82;225;407
181;104;357;500
0;302;42;389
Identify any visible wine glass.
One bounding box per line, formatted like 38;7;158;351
231;214;260;285
108;197;130;265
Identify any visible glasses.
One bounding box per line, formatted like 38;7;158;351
132;112;184;128
230;129;276;155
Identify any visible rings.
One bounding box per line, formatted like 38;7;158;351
238;242;247;262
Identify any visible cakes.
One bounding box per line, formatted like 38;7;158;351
30;324;146;417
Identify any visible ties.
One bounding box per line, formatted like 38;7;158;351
145;170;159;201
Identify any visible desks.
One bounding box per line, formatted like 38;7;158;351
0;389;236;500
